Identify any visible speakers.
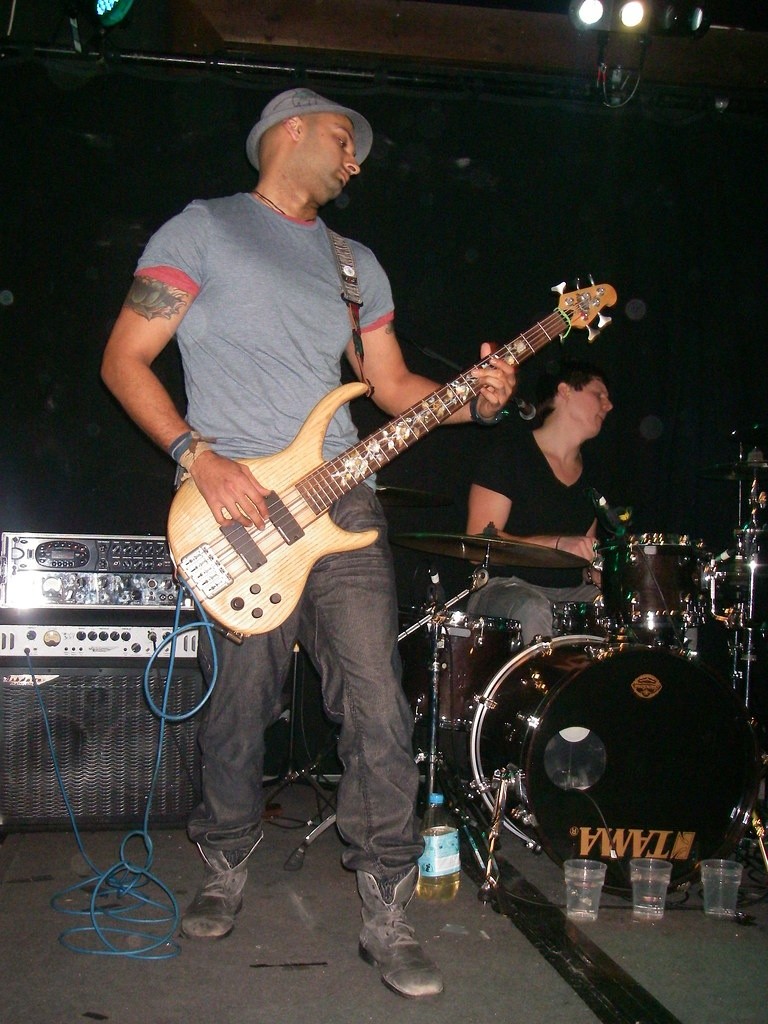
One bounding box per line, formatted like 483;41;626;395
0;664;202;832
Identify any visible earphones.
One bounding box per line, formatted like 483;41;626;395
565;388;571;396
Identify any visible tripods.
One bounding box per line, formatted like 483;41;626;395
284;543;520;915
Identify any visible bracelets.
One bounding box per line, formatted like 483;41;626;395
554;535;563;552
168;429;212;489
470;396;502;427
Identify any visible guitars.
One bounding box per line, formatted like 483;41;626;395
164;267;619;639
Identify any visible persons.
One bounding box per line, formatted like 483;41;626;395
466;354;626;661
101;86;518;996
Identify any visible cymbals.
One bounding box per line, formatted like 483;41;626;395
708;462;768;481
375;485;454;507
388;532;591;569
715;562;768;578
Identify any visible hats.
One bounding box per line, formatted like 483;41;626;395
246;88;373;173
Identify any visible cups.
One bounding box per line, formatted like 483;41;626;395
629;858;673;919
564;859;609;920
700;859;743;917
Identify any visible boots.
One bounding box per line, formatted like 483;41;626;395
356;866;444;999
180;829;264;941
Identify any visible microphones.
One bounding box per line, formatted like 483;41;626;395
714;547;737;563
592;488;626;537
517;398;537;421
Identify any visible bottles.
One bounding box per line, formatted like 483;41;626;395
414;792;460;902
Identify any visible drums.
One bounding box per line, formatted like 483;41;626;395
398;603;525;732
550;601;687;647
592;533;708;617
468;634;761;893
